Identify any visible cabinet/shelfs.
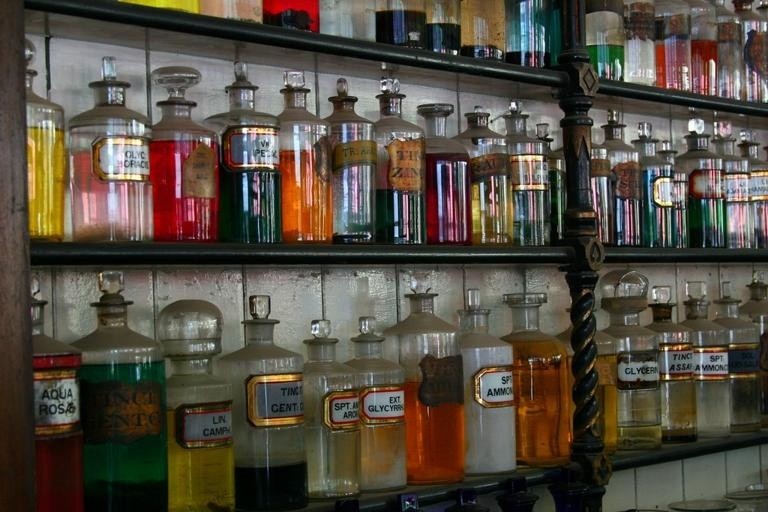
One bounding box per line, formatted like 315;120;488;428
1;1;767;511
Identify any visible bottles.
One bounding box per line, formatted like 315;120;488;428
302;320;360;499
219;295;310;511
70;268;169;511
120;0;566;69
276;67;334;243
28;272;84;510
148;65;222;242
345;316;408;492
416;103;470;243
373;76;426;244
501;103;551;244
594;269;768;512
382;288;467;483
321;76;376;246
584;0;768;105
499;292;574;470
23;38;68;243
201;55;281;244
66;55;155;244
534;123;566;244
156;299;237;511
454;288;517;475
592;107;768;249
448;103;514;244
